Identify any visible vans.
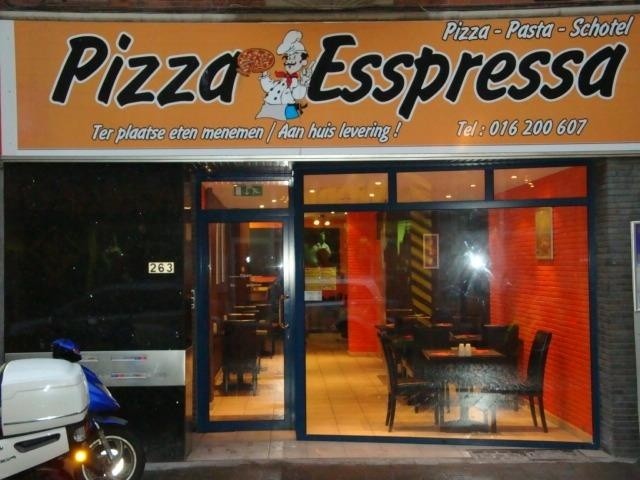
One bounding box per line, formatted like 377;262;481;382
262;277;383;338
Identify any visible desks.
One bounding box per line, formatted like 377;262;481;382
249;275;277;284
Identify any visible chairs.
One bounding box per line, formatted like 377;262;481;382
376;307;553;432
221;302;274;394
245;283;270;302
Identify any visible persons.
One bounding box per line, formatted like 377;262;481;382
311;232;331;265
265;266;284;337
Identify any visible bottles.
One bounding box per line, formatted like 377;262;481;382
458;344;465;356
464;344;472;356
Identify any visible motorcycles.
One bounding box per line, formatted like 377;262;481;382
0;338;146;480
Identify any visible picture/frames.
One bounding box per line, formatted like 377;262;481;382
422;233;440;269
535;206;554;261
